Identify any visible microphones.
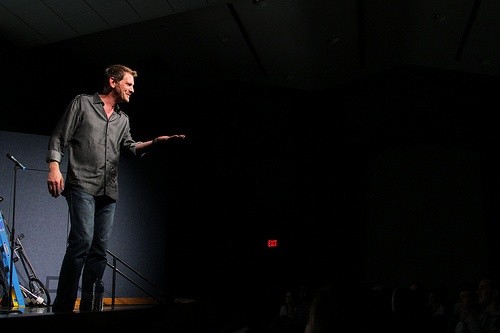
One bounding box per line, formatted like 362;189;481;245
119;86;125;95
7;153;26;169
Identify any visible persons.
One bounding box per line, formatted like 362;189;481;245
278;276;500;333
45;64;187;313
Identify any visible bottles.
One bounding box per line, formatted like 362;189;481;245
95;280;104;312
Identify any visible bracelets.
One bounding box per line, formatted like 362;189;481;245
153;138;160;146
